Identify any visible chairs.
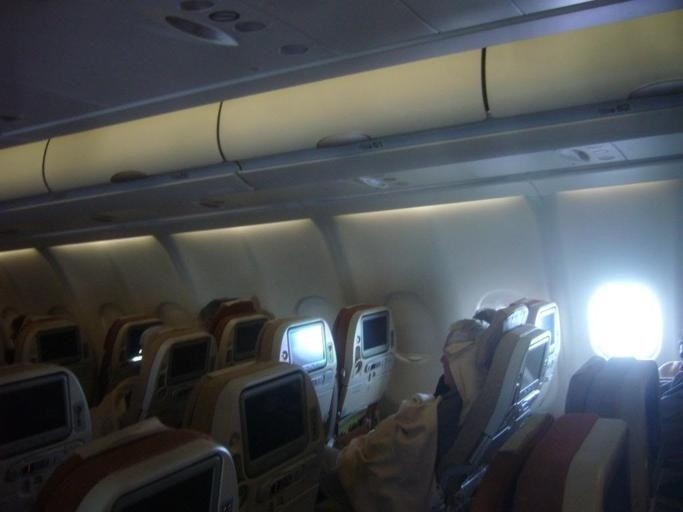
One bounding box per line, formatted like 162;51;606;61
490;301;560;377
0;302;81;369
332;303;396;430
471;412;627;512
0;365;95;512
91;314;162;400
181;361;325;512
32;417;239;512
214;308;274;371
253;315;338;447
89;316;215;435
566;356;683;512
321;325;552;512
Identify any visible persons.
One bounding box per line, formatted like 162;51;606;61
309;320;486;512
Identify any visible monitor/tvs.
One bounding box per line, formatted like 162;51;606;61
114;456;220;512
232;318;267;356
514;337;550;401
239;374;307;479
358;311;392;357
36;326;80;362
123;321;163;363
0;374;71;459
287;321;327;373
539;309;556;353
165;336;211;386
